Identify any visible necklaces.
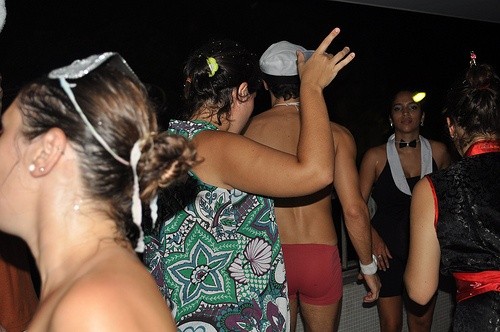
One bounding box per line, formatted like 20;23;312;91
274;102;299;111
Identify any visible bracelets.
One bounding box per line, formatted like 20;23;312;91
359;255;377;275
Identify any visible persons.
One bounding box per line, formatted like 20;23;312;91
359;85;453;332
0;51;182;332
238;40;382;332
402;64;500;332
132;27;355;332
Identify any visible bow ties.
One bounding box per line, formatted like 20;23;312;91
399;139;417;148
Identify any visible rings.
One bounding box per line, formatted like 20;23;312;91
378;255;381;258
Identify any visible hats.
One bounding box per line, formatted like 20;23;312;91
259;41;306;76
